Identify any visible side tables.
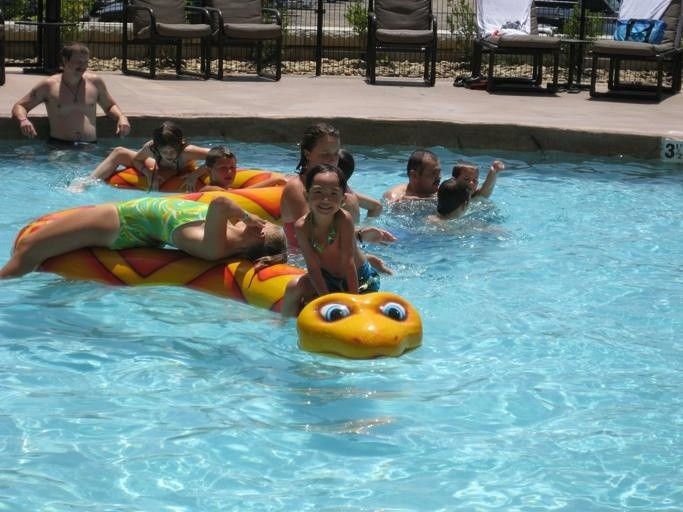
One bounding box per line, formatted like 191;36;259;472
15;20;76;75
558;40;595;94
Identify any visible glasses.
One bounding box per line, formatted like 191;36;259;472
312;226;337;253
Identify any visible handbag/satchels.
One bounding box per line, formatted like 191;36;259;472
613;18;666;43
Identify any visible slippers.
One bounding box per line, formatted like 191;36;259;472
464;76;487;87
454;73;467;87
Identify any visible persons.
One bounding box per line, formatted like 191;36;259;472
11;42;131;141
279;122;397;276
333;149;384;225
90;120;213;194
425;178;507;238
0;195;288;280
197;144;289;193
450;159;506;198
280;163;381;318
382;148;441;206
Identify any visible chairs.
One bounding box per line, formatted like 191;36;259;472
590;0;683;105
472;0;560;95
367;0;437;87
185;1;280;79
120;1;214;82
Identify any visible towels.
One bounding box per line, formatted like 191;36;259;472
617;0;673;21
475;0;533;41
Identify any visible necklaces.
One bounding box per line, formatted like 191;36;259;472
60;75;82;103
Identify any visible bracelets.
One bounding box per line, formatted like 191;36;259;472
244;209;249;222
358;230;363;243
